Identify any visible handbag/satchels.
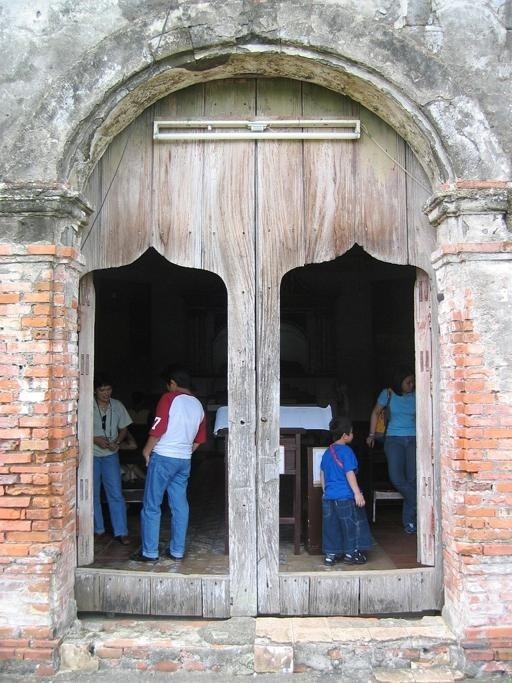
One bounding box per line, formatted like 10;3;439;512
376;406;390;438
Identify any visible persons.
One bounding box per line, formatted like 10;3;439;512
127;364;208;564
318;413;374;565
365;364;416;534
94;373;134;546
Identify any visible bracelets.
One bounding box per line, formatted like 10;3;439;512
115;439;121;445
368;431;375;437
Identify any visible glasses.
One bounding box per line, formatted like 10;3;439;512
102;414;106;430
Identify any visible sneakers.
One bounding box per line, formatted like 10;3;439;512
166;548;184;560
405;523;417;534
130;547;158;561
324;553;338;565
343;549;367;564
120;535;130;544
